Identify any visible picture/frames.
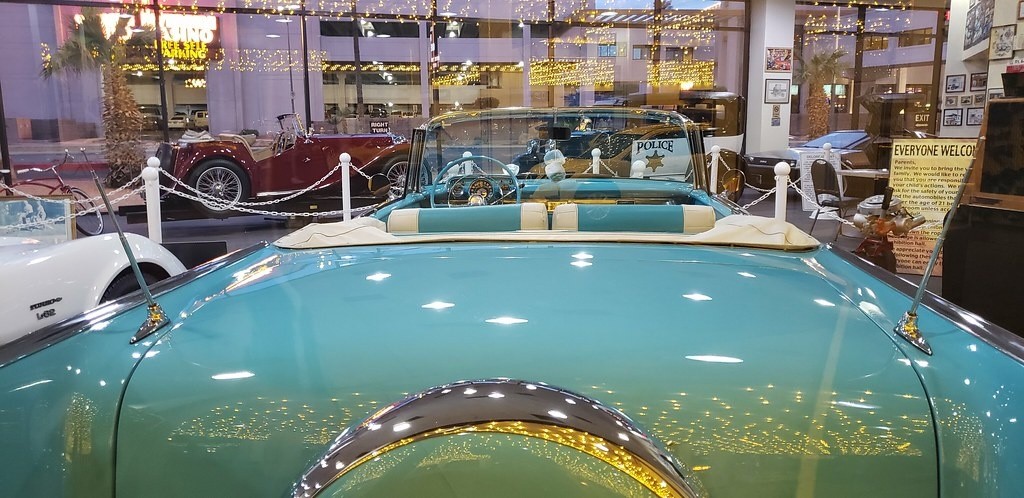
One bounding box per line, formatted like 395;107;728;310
959;95;973;106
765;46;792;73
943;108;964;126
988;24;1017;60
946;74;966;93
1017;1;1024;19
944;95;959;107
974;94;986;104
966;107;985;125
765;79;790;103
970;72;988;91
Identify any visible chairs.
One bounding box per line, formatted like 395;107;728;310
386;203;715;235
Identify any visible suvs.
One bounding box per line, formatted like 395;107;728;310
139;114;433;222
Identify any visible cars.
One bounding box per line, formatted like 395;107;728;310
0;230;189;344
507;129;613;184
742;129;877;200
1;109;1024;497
532;121;715;198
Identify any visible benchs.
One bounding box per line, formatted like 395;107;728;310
219;133;254;160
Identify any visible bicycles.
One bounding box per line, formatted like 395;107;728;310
1;150;105;235
